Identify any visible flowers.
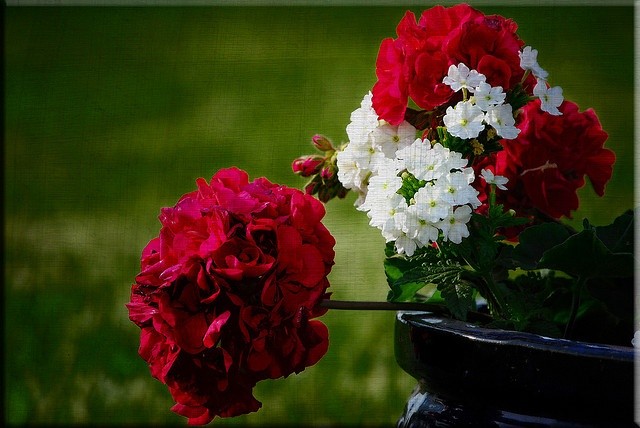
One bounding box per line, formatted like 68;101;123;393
124;2;635;427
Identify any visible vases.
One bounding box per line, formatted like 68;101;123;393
394;309;636;427
